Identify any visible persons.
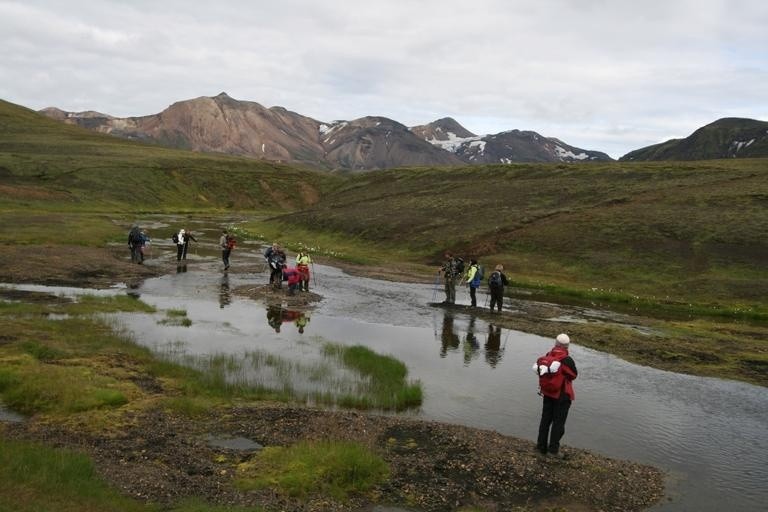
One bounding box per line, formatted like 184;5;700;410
438;250;458;305
487;264;509;312
462;259;480;309
126;223;199;264
219;231;231;270
264;242;314;297
532;333;578;459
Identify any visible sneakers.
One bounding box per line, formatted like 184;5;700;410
224;264;230;270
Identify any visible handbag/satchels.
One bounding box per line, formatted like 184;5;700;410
471;280;480;288
455;257;465;273
489;271;502;288
172;234;179;243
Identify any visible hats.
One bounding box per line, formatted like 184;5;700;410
555;333;570;347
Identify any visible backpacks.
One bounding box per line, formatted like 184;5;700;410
472;264;484;281
537;351;568;391
222;235;236;249
